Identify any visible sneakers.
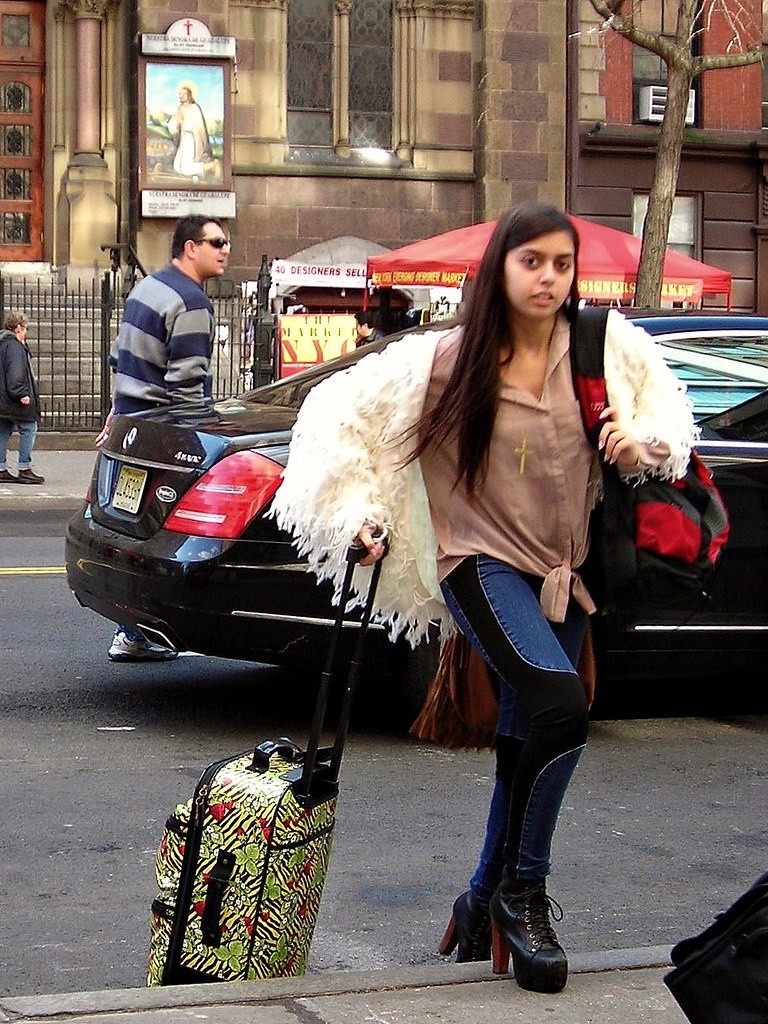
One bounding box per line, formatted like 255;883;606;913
108;624;178;660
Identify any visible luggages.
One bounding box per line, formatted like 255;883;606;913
145;539;389;987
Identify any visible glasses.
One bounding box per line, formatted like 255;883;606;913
21;324;29;330
195;238;232;251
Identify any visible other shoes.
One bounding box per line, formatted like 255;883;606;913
19;469;44;483
0;469;19;482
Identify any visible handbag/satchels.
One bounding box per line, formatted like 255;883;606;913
663;869;768;1024
583;459;729;616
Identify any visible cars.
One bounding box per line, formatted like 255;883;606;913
64;306;768;722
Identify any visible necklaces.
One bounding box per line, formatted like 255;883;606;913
513;395;542;476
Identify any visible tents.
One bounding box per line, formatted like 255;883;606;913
365;209;732;313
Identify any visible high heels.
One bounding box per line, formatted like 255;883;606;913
437;888;493;963
494;864;568;994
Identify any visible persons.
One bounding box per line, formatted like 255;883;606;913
355;311;386;347
96;213;230;659
0;312;45;484
278;200;700;994
